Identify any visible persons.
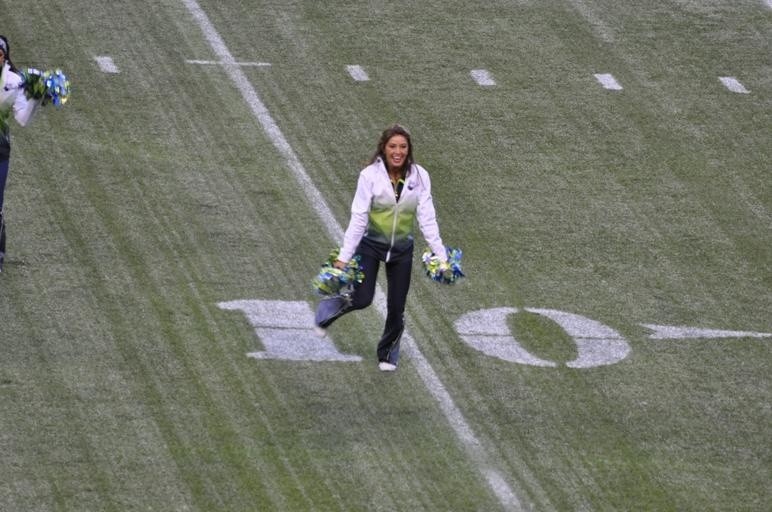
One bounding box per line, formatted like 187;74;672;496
315;124;454;371
0;35;47;272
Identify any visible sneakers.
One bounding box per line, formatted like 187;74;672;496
379;360;396;371
312;316;328;338
